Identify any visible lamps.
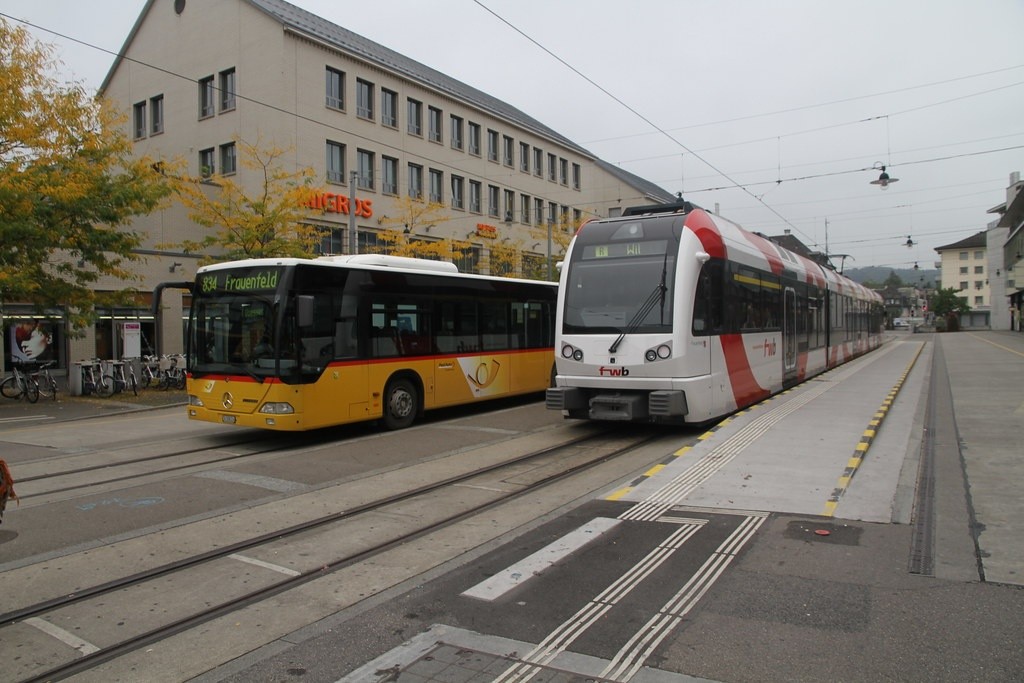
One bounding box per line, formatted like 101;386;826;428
497;211;519;230
910;263;922;271
397;223;416;240
902;236;918;248
870;166;899;190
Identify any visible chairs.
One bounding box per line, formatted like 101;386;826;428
372;325;525;357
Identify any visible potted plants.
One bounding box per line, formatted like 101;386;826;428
933;286;970;332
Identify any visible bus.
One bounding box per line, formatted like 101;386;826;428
545;189;888;429
152;254;560;434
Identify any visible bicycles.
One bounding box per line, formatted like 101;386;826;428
0;353;189;406
